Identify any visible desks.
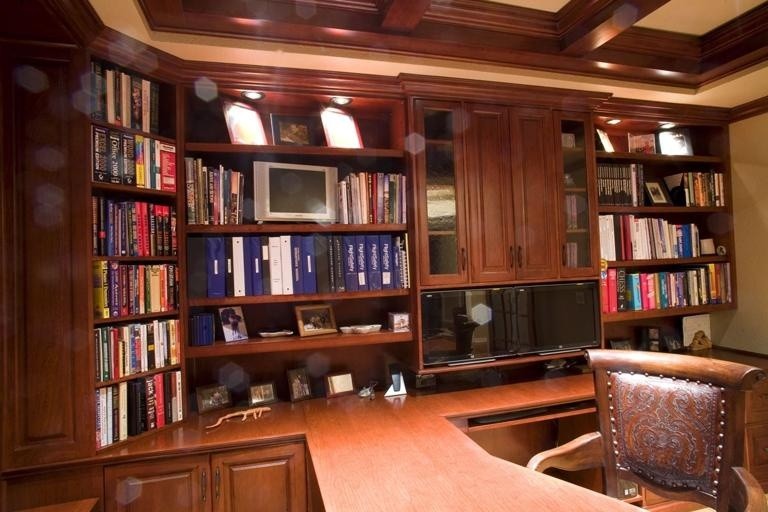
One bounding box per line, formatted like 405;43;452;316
100;350;768;512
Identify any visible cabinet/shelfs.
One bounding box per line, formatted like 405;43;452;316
408;96;605;374
90;51;185;453
104;443;306;511
181;83;417;359
593;113;738;323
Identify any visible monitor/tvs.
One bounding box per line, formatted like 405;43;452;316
420;281;601;368
252;160;337;224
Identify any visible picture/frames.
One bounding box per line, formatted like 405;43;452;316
246;380;278;408
656;129;693;157
296;303;338;338
195;382;232;414
285;364;313;402
320;106;365;149
323;369;357;399
270;113;316;146
220;97;268;145
644;179;672;207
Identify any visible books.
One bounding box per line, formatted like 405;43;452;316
96;370;183;450
91;62;160;134
93;260;179;319
91;127;177;194
185;157;245;225
92;196;177;256
94;319;180;382
189;313;217;345
598;163;645;207
186;235;410;299
663;172;725;206
598;216;701;262
338;172;407;225
600;263;732;313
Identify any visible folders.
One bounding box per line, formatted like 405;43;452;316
232;237;245;296
243;237;252;296
268;237;282;295
280;236;294;295
261;237;270;295
225;237;234;296
379;235;393;289
366;236;382;291
328;236;335;293
251;237;263;295
355;236;368;291
188;237;207;298
343;236;358;292
302;237;316;293
290;236;304;294
207;237;225;298
315;237;330;293
332;236;346;292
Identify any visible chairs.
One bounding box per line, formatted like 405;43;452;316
526;349;767;512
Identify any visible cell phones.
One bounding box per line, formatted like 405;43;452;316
257;328;293;339
